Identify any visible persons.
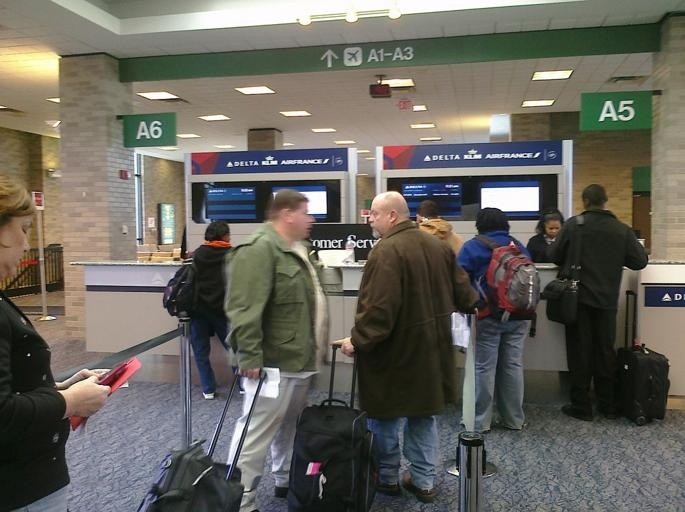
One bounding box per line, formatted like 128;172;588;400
546;183;650;422
415;198;466;259
526;206;564;264
456;207;531;435
331;189;480;504
223;188;331;512
1;172;111;512
189;219;246;400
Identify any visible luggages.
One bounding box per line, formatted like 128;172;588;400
138;369;266;512
286;344;379;510
616;290;669;425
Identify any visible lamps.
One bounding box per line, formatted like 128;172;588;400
293;9;403;28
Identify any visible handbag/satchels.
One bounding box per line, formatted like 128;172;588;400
543;280;581;324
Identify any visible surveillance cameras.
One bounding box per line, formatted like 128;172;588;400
369;84;392;98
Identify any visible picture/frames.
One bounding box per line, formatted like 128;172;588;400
158;202;176;245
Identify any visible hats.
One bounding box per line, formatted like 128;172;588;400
475;208;508;230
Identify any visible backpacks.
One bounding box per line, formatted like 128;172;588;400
472;235;539;339
164;251;201;317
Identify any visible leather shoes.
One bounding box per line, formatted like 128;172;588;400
596;406;620;421
561;403;593;422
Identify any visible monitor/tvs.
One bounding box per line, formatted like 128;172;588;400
204;184;261;223
400;180;463;216
478;179;543;220
270;183;329;221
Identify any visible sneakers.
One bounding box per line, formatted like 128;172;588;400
402;469;437;503
374;473;401;495
201;390;215;401
